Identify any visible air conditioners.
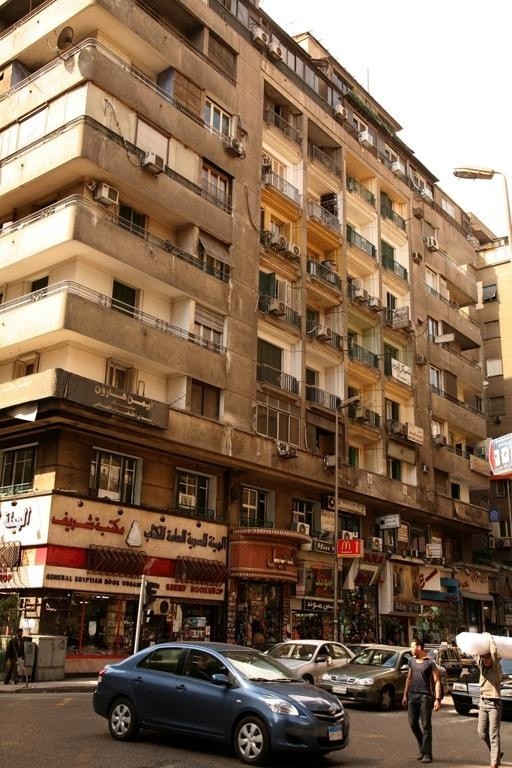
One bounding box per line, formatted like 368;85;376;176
354;285;386;313
223;135;248;160
294;519;310;537
252;26;285;63
420;185;436;205
270;230;301;259
141;147;165;177
391;158;407;179
358;127;378;150
93;180;120;207
369;535;385;553
424;233;442;251
354;403;372;424
334;103;350;122
315;325;334;340
337;528;356;543
267;296;288;317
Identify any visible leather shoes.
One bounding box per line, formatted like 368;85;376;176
422;754;433;763
416;746;423;760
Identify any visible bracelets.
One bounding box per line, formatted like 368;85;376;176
435;697;442;701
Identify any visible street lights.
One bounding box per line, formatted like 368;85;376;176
333;396;361;641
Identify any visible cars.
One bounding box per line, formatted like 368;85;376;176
263;638;511;716
92;642;350;763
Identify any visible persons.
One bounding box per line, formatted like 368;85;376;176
470;631;504;768
401;638;442;762
3;629;26;686
